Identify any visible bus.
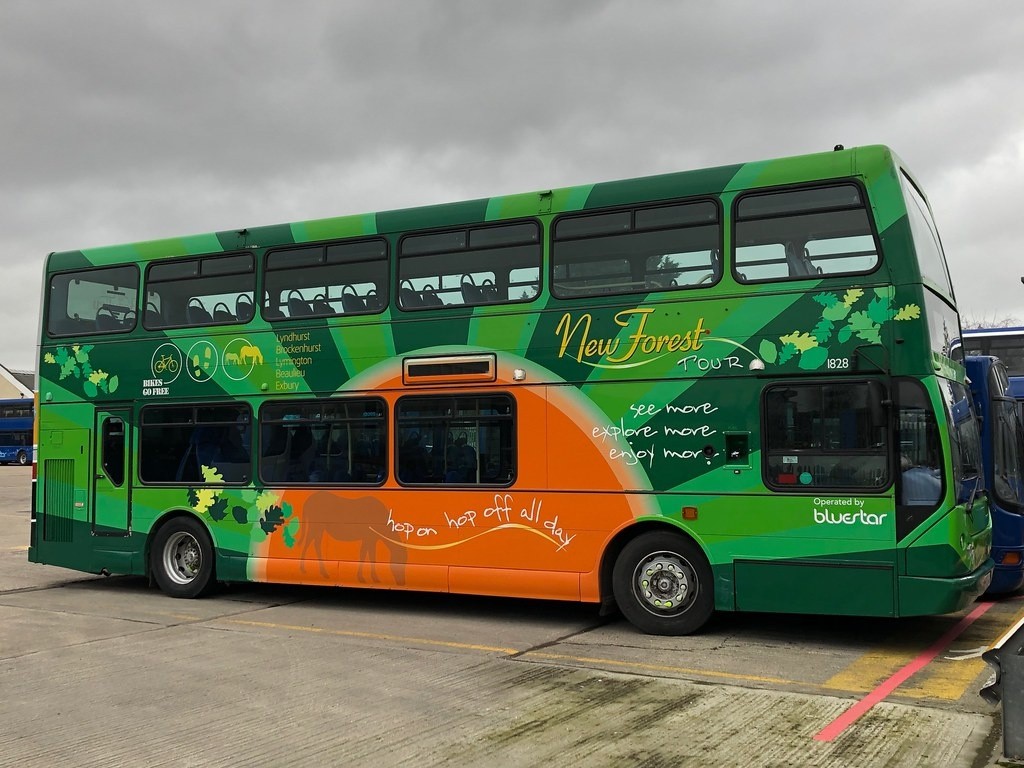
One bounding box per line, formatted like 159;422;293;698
962;324;1024;507
0;397;35;466
26;144;1000;638
900;353;1024;601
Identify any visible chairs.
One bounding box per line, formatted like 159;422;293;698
709;248;747;282
785;241;824;275
73;270;505;336
211;454;478;484
